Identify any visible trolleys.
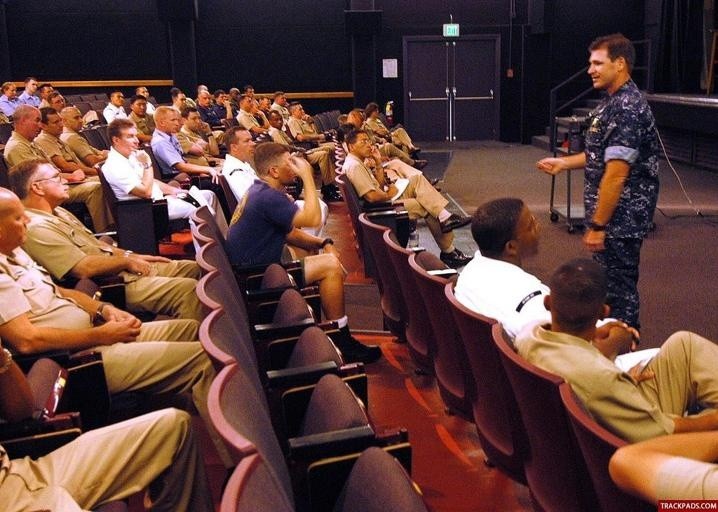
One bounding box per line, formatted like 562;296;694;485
548;115;593;236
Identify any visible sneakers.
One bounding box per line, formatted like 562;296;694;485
323;192;342;201
408;147;420;154
321;186;340;192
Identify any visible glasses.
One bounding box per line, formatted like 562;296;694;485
361;140;372;144
35;173;61;185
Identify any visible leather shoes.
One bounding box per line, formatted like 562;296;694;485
441;213;471;233
429;178;438;185
414;160;427;168
440;248;472;267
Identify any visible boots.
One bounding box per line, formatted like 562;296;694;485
328;325;380;363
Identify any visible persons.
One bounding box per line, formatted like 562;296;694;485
606;429;716;511
0;77;474;363
0;341;216;511
452;198;552;342
0;187;234;473
533;32;661;328
518;252;718;440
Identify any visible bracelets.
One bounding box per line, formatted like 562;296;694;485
0;347;13;375
587;221;607;233
